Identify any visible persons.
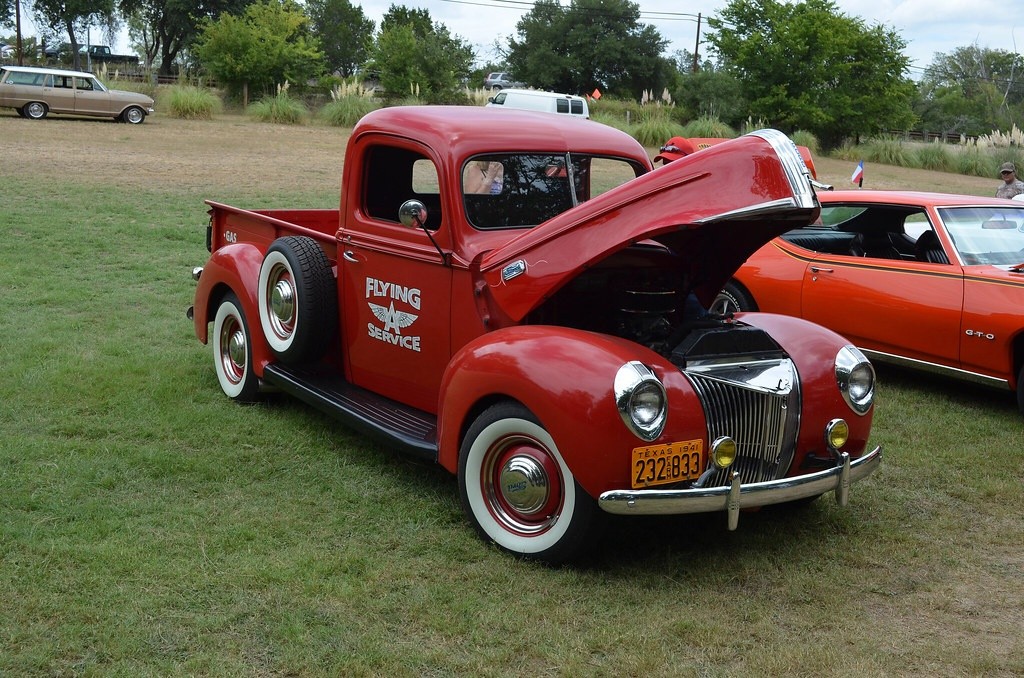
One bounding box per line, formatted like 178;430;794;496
464;161;503;194
994;162;1024;199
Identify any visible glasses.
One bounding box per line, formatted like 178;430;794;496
659;145;688;156
1001;171;1012;175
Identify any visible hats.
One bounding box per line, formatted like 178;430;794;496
1000;162;1016;174
653;136;695;162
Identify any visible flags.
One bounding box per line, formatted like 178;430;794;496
852;160;863;183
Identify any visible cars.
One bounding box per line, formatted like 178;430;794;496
707;189;1024;411
138;61;157;69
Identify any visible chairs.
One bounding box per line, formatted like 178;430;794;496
914;230;956;264
847;233;903;261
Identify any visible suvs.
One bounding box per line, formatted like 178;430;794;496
485;73;525;89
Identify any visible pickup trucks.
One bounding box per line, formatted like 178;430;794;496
0;42;138;70
186;106;881;557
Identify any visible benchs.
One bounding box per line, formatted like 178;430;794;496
780;234;886;256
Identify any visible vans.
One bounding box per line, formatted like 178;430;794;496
0;66;155;124
485;90;589;120
